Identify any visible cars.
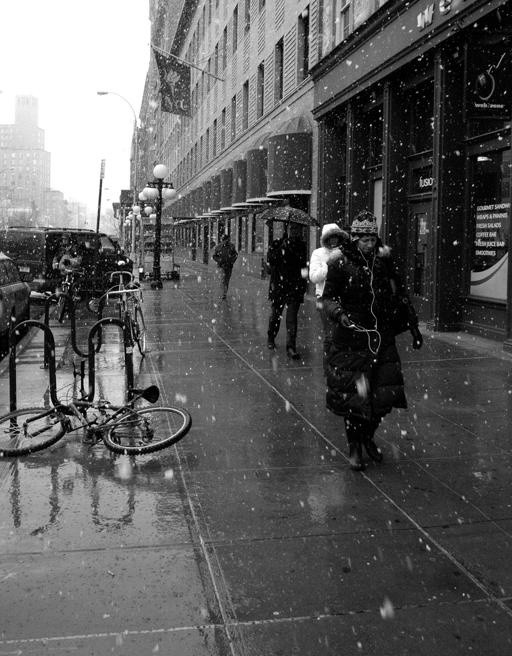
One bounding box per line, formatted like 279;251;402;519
0;250;32;346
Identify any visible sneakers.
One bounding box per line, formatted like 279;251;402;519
365;438;383;462
267;337;274;349
288;347;299;359
350;449;365;471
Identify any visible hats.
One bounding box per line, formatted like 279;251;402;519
349;210;379;243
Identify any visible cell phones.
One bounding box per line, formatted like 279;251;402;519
346;320;356;328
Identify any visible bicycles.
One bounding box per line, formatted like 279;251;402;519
56;266;104;324
0;357;194;458
106;269;150;357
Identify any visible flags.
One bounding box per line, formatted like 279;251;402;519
151;49;194;118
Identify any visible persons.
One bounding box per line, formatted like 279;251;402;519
265;224;307;360
307;222;351;383
212;232;239;302
318;209;424;473
51;233;85;303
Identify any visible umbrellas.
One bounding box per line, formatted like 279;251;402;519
259;206;321;246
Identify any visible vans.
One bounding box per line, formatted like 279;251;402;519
0;225;135;300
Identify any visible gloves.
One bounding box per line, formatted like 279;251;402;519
340;312;354;329
411;327;423;349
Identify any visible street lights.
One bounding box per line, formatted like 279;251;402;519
119;161;180;292
97;90;139;206
